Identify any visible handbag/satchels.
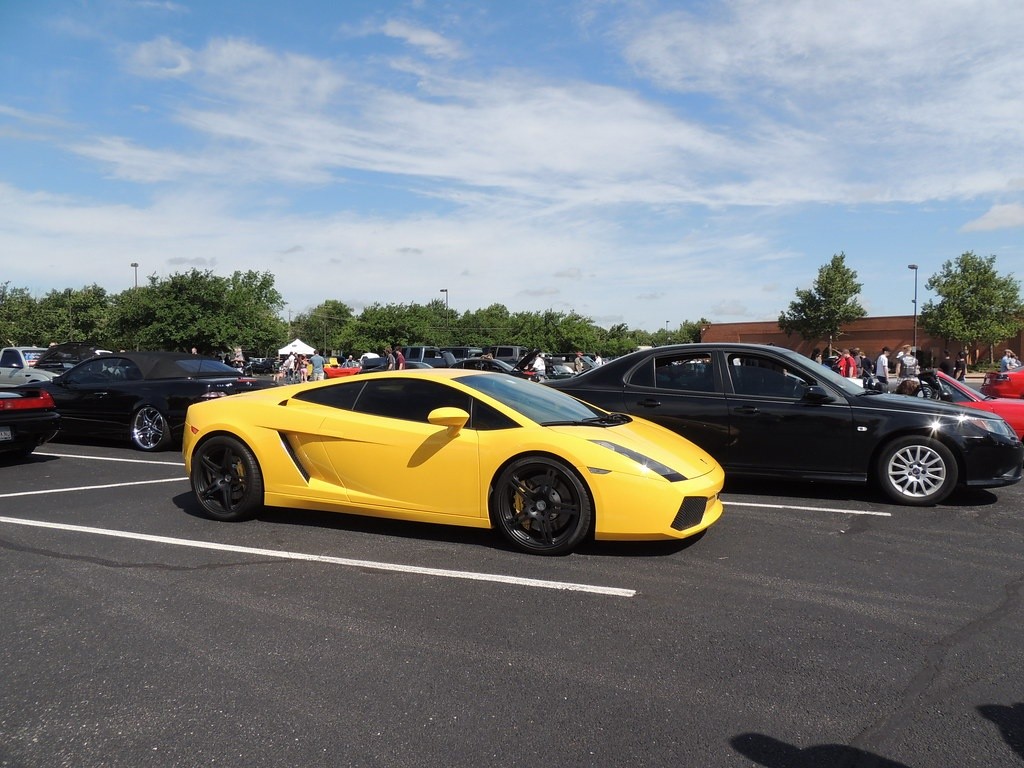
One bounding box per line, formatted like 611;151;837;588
963;364;968;375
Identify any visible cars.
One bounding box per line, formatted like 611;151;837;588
0;343;99;388
540;344;1024;507
861;365;1024;445
0;384;63;460
20;352;278;452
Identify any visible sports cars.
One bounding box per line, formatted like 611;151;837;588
181;368;727;557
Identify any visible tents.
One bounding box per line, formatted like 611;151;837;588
278;339;316;360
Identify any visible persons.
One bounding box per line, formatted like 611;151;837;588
690;358;709;377
1001;349;1021;372
394;346;405;370
273;350;325;383
594;352;603;367
224;354;231;365
575;352;583;374
530;349;546;372
812;344;968;397
385;347;395;371
346;355;353;367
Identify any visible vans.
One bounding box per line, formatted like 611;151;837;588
302;345;596;382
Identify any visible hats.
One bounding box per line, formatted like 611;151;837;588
577;352;583;356
882;346;892;352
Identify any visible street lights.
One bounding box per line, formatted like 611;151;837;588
440;290;448;327
908;264;918;347
